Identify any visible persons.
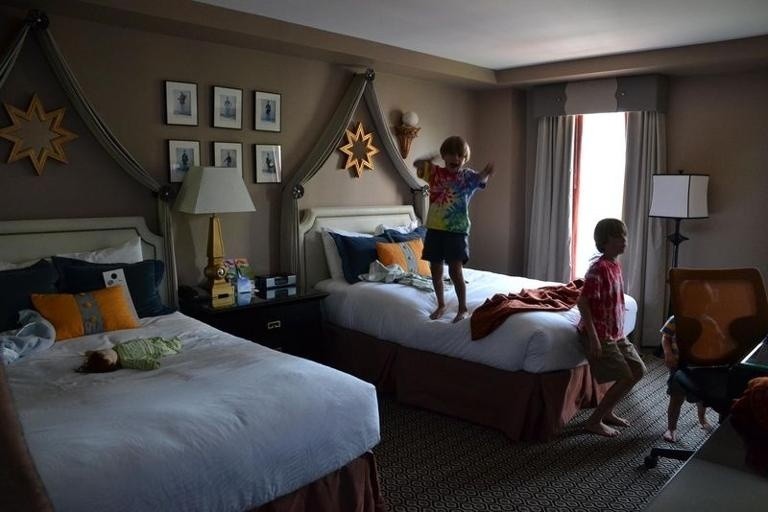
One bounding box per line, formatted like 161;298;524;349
224;152;232;167
73;334;184;374
181;150;188;166
577;218;647;436
413;136;493;323
659;280;726;442
224;96;232;116
176;91;187;112
265;100;272;119
266;153;272;172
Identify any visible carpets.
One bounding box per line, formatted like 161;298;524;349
374;352;731;511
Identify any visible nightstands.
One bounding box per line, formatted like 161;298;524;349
179;283;331;363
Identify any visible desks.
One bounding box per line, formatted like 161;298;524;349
726;331;768;411
642;415;768;512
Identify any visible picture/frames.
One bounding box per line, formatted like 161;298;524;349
252;89;281;132
168;139;202;184
211;141;244;181
164;80;199;127
211;84;244;130
254;143;283;184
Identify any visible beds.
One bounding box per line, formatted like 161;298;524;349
0;215;383;512
299;204;638;444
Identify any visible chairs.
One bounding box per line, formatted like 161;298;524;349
645;268;768;469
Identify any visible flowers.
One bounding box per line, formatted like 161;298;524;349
221;258;251;279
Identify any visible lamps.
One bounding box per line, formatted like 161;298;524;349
391;110;421;159
171;166;256;308
648;170;710;358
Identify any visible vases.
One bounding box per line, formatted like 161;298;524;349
231;277;253;293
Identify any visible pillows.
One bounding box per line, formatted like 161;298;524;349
1;258;59;332
29;283;142;340
328;231;390;285
56;235;144;264
376;237;432;277
377;219;419;235
50;256;178;319
383;225;427;244
319;224;376;279
